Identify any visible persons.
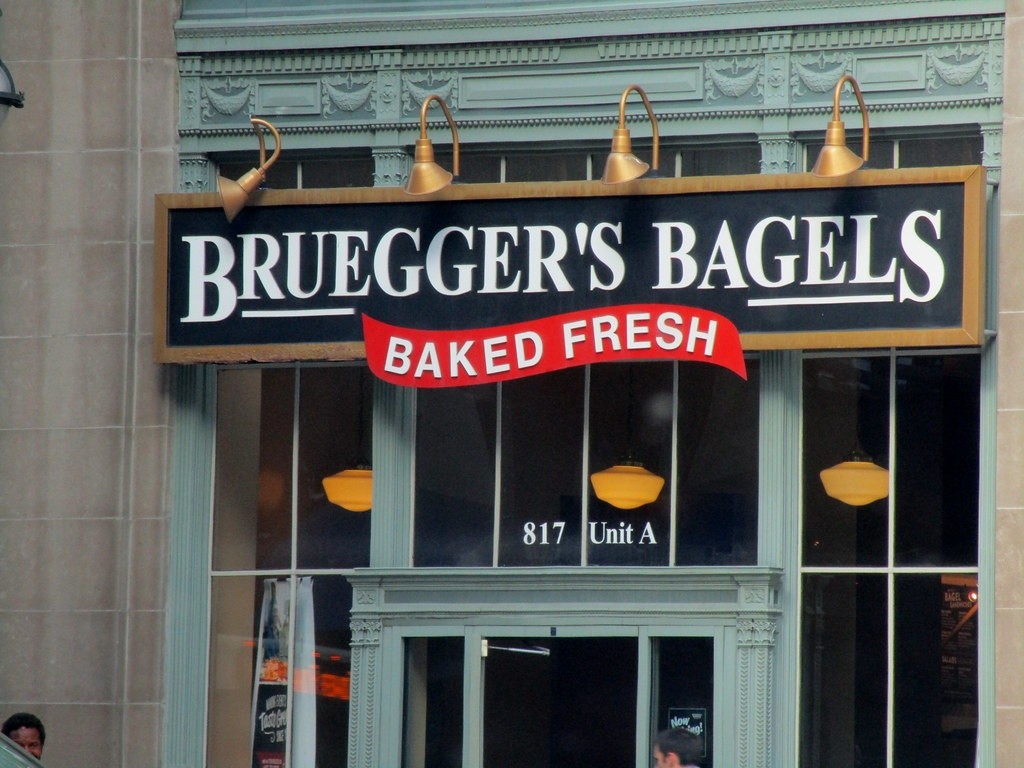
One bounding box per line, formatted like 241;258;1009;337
1;712;45;761
652;727;703;768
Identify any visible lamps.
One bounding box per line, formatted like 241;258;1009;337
819;451;890;506
811;74;870;177
217;118;282;223
601;84;660;184
592;454;665;509
403;94;459;195
322;453;372;511
